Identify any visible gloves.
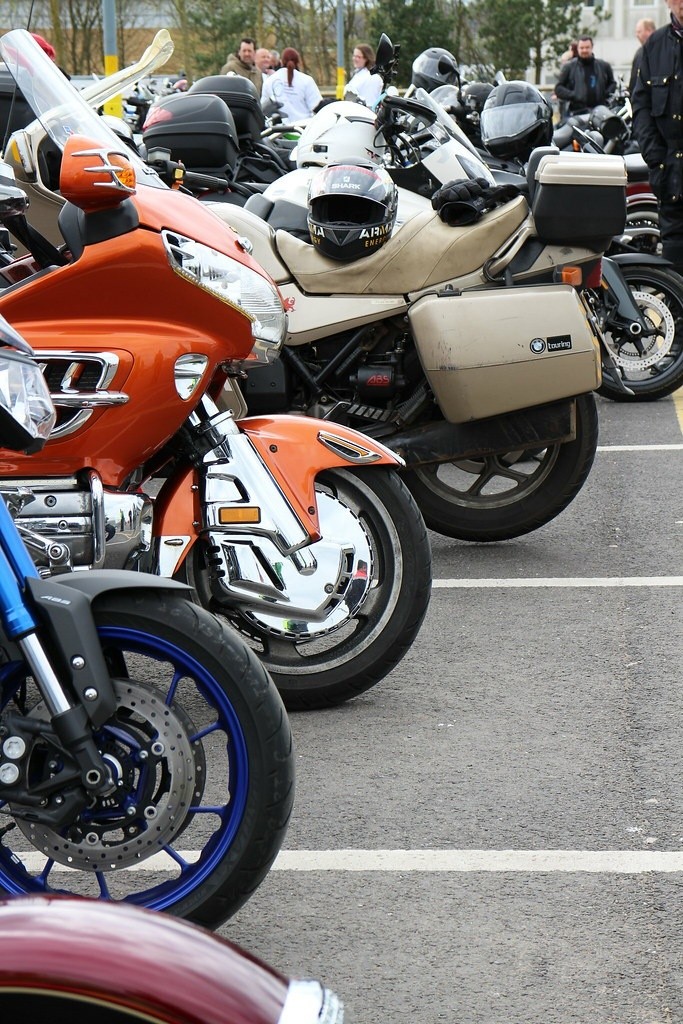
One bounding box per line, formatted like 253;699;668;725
431;179;522;227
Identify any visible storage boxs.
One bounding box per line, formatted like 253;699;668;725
408;285;603;420
533;150;628;240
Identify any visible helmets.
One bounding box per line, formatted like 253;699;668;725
480;80;554;159
465;82;494;116
306;157;399;261
412;47;461;93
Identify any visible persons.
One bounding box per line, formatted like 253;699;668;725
630;0;683;358
551;35;616;113
627;17;658;99
219;37;386;127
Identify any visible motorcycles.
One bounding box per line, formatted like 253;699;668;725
0;29;683;542
1;28;429;713
0;493;296;933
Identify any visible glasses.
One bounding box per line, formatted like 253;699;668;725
353;55;367;60
